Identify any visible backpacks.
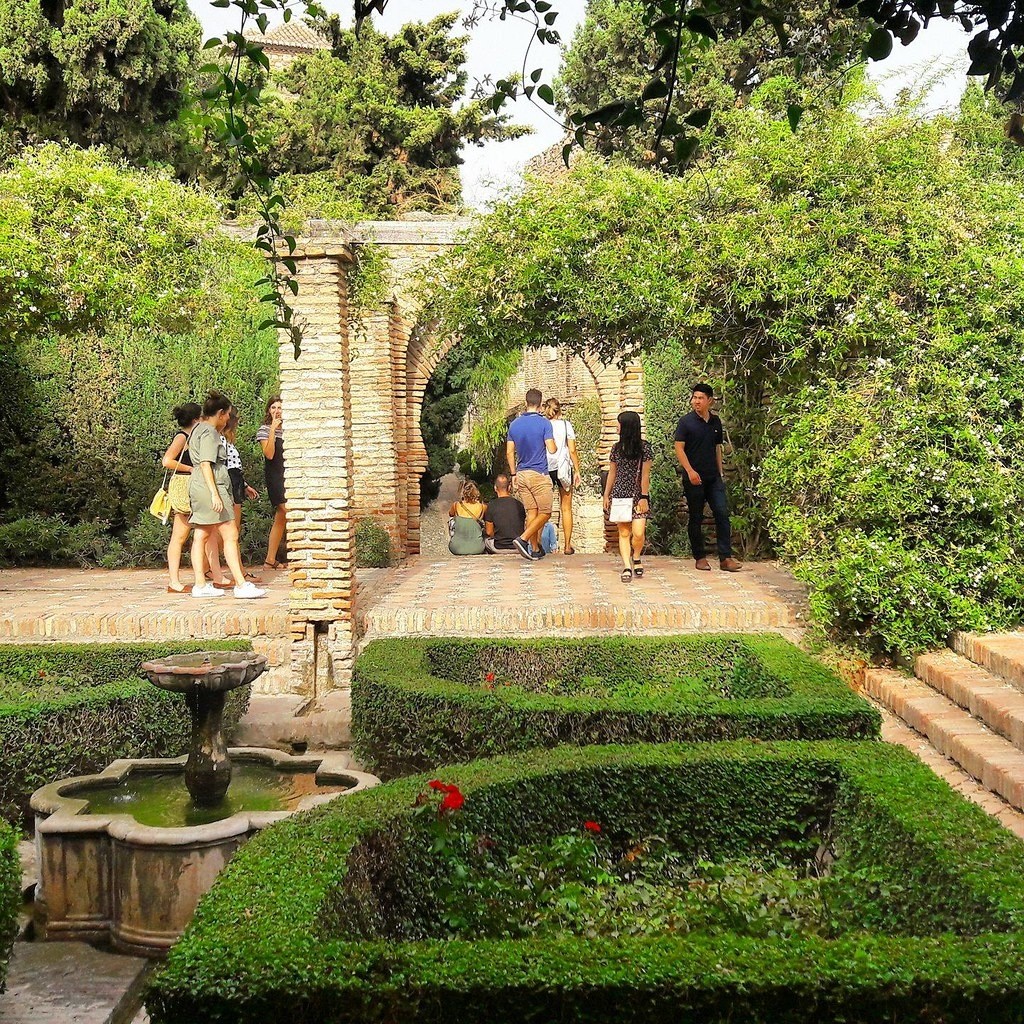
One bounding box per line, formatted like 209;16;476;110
169;474;192;514
150;488;171;525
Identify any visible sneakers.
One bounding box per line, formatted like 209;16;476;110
233;583;266;599
696;558;711;570
192;583;225;597
720;558;743;570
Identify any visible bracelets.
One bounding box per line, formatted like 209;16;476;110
244;485;249;489
510;472;516;476
639;495;649;499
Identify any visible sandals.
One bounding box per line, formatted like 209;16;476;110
204;569;212;580
632;557;643;576
262;561;287;571
245;572;261;582
621;568;632;583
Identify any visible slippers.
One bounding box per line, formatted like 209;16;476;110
564;547;574;554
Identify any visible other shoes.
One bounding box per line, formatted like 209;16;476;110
513;537;539;560
213;575;235;588
532;551;543;558
168;586;192;593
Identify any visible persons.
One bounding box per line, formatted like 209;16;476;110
161;402;235;594
448;478;488;555
506;388;557;560
257;395;289;571
603;411;654;583
673;384;744;572
187;389;267;599
535;397;582;555
199;406;264;582
540;522;559;554
485;473;526;555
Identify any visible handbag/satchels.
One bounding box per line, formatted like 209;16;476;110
448;518;456;537
609;497;633;523
557;445;573;492
